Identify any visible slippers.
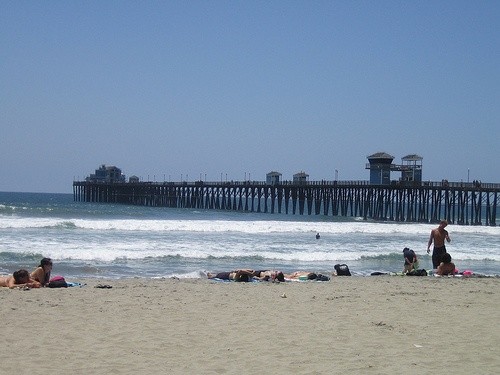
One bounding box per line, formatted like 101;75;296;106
96;284;112;288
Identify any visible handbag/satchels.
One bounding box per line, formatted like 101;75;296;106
48;280;67;288
406;268;426;276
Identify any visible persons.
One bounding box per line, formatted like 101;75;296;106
428;253;456;277
316;233;320;239
252;270;284;282
206;270;250;282
402;247;415;273
1;270;40;289
30;257;52;284
428;222;450;268
284;271;317;280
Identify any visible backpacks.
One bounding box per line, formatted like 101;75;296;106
334;262;351;276
